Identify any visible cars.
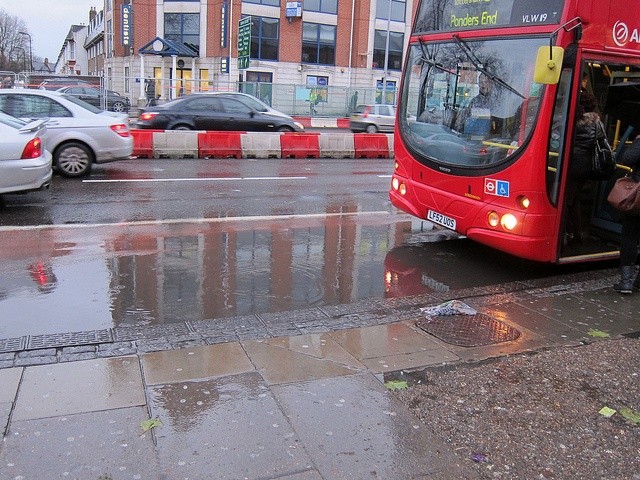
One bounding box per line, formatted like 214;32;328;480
138;96;304;133
349;106;416;134
53;86;130;113
0;111;52;194
0;89;134;177
161;93;293;119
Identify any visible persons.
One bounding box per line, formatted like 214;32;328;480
143;75;155;107
310;89;319;115
614;134;640;294
263;93;270;106
569;104;609;245
347;91;358;113
463;71;502;128
581;70;594;103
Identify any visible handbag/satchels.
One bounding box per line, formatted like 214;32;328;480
606;170;639;214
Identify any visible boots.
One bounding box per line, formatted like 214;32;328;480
611;263;634;295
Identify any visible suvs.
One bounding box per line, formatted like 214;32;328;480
39;80;100;89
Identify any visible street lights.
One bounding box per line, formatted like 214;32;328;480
19;32;32;72
16;47;26;88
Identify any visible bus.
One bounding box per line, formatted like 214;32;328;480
390;0;640;265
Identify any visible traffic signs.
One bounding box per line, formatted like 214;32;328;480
238;17;251;70
